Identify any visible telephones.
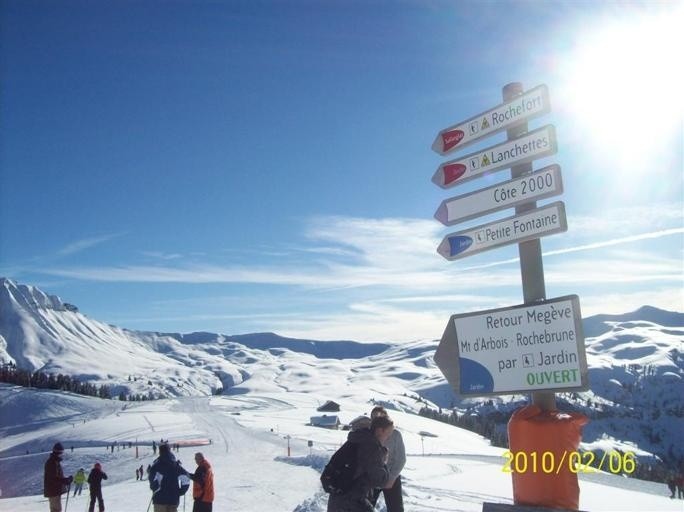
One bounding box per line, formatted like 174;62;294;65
430;83;568;262
432;293;590;399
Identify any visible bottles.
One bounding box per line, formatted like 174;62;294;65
94;462;101;470
52;442;64;454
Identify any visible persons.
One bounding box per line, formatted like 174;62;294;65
185;452;214;512
42;440;71;512
136;464;153;480
72;466;84;496
326;414;394;512
364;406;404;511
86;462;107;512
147;444;189;512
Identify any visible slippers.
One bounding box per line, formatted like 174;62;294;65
320;439;365;494
